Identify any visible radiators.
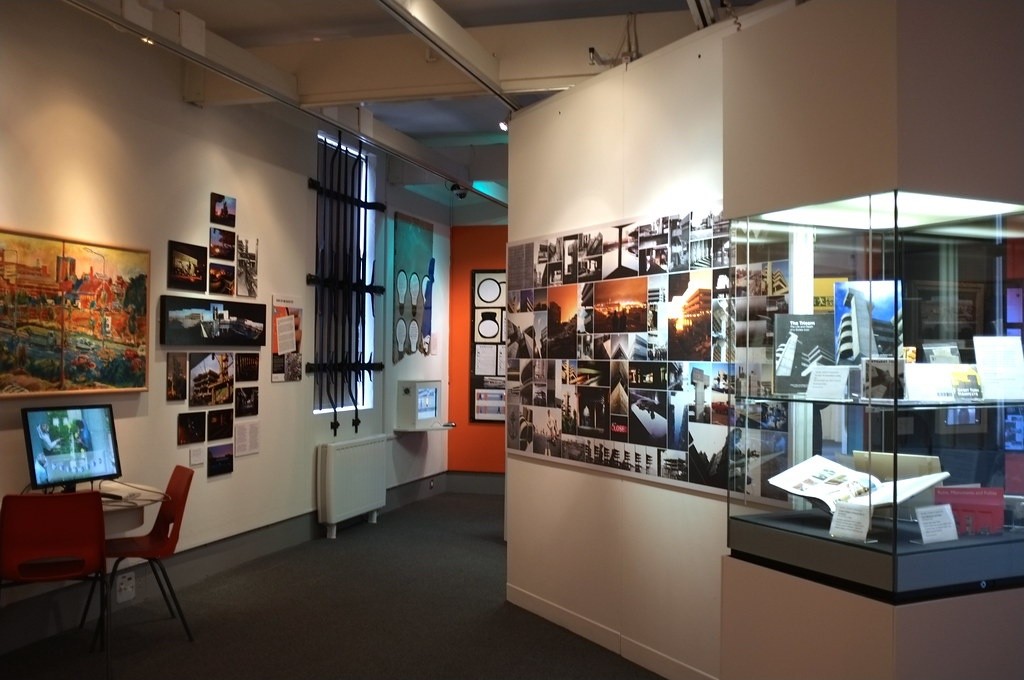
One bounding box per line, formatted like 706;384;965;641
316;432;390;539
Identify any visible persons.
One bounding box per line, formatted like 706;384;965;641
73;418;94;466
725;428;757;495
545;409;556;443
39;424;64;457
35;454;50;485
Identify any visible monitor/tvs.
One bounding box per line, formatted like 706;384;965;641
21;404;122;501
418;388;437;420
935;408;988;434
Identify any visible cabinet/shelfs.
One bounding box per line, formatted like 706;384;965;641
725;188;1024;607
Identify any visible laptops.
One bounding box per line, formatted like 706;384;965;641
852;449;944;520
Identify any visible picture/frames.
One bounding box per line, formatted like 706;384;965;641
0;228;151;399
167;238;208;292
160;296;266;346
469;269;507;424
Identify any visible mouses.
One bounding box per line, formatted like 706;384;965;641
443;422;456;427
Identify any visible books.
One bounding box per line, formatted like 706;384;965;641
768;454;950;517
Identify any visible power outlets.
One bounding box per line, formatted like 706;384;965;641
114;571;136;604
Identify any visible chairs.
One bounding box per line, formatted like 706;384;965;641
1;491;107;658
74;464;194;656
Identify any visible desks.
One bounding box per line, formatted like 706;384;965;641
19;477;165;538
